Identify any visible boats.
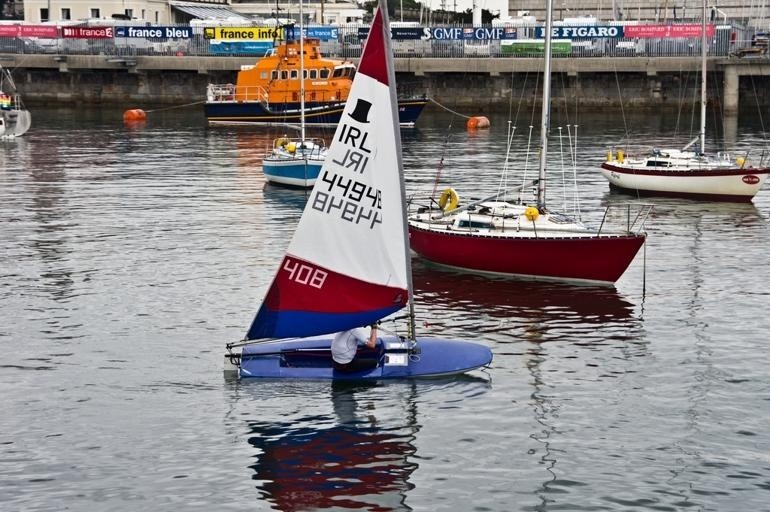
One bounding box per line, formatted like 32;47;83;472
203;2;430;131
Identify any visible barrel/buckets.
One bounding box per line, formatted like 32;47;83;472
466;116;491;130
122;108;146;121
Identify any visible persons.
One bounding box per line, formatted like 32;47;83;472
331;325;378;373
207;79;214;92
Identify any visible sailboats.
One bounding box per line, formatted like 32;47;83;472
408;0;650;295
604;1;770;202
222;2;492;382
261;3;335;194
0;59;31;145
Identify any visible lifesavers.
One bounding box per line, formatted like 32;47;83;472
277;138;291;150
616;150;624;165
438;188;459;213
